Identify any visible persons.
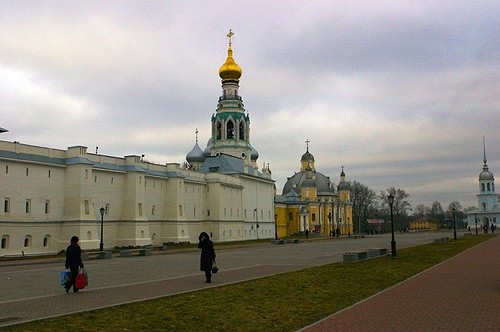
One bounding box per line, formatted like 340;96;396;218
64;236;84;294
197;232;216;283
303;222;498;240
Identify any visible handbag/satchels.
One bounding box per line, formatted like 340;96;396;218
60;268;71;286
84;269;88;286
76;267;85;289
212;260;219;273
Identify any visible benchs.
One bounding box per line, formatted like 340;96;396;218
120;248;151;257
343;247;389;261
83;251;112;261
274;239;312;245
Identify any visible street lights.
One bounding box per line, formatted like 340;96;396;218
99;206;105;250
387;193;397;256
452;207;457;241
